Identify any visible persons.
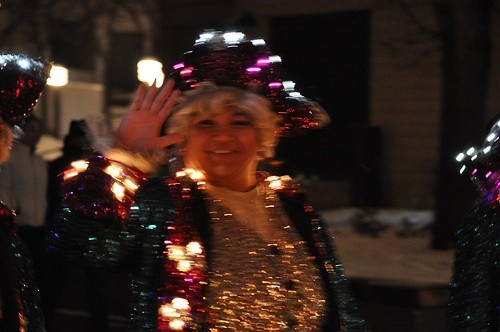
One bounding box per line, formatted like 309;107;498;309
0;55;97;332
47;30;366;332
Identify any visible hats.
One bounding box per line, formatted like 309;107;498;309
168;30;330;136
0;53;54;130
